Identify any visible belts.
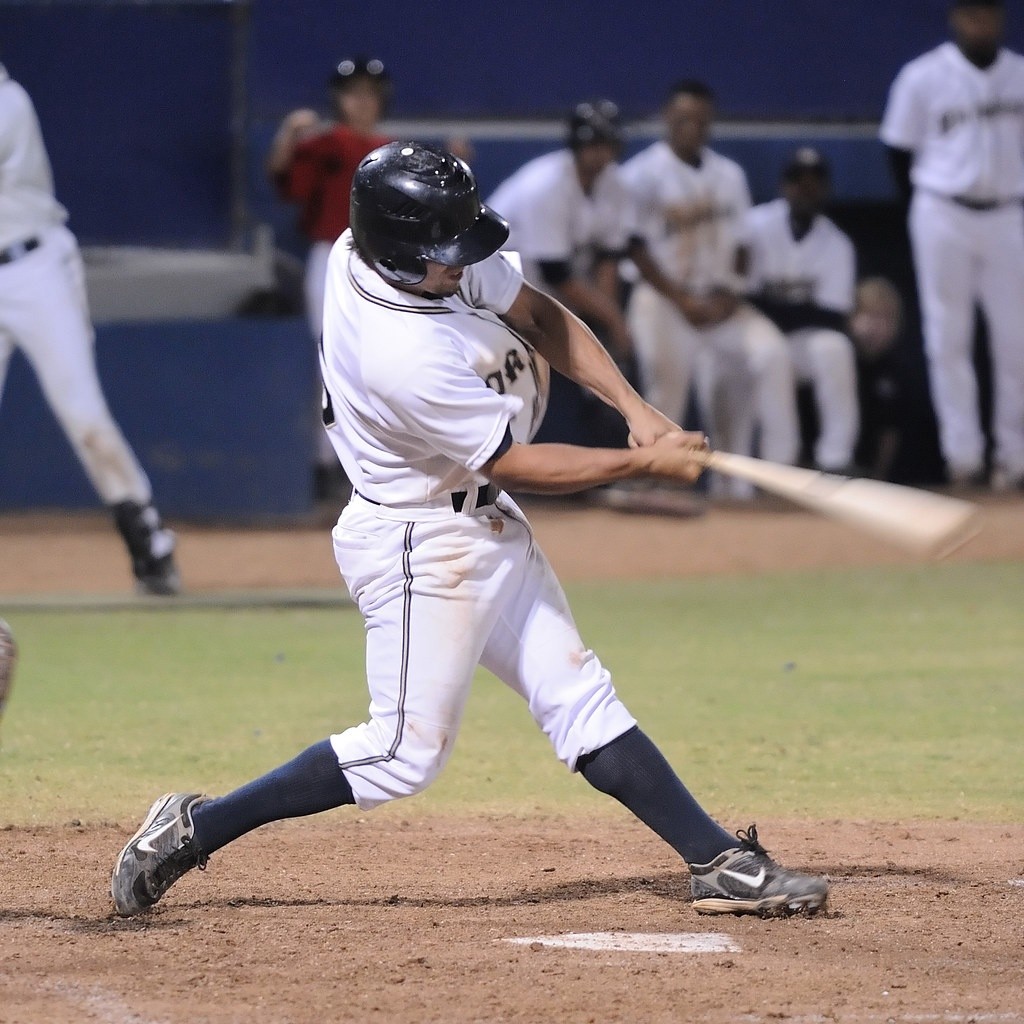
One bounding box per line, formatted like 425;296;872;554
951;193;994;211
0;235;40;266
355;483;503;513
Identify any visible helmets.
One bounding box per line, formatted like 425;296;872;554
352;141;510;284
567;98;622;148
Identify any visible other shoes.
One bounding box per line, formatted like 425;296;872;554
113;500;181;595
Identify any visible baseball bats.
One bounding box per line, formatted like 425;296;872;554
620;422;994;574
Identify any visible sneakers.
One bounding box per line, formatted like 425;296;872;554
689;824;826;916
110;791;210;920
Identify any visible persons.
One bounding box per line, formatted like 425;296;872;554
0;64;181;596
107;138;827;921
265;0;1023;496
